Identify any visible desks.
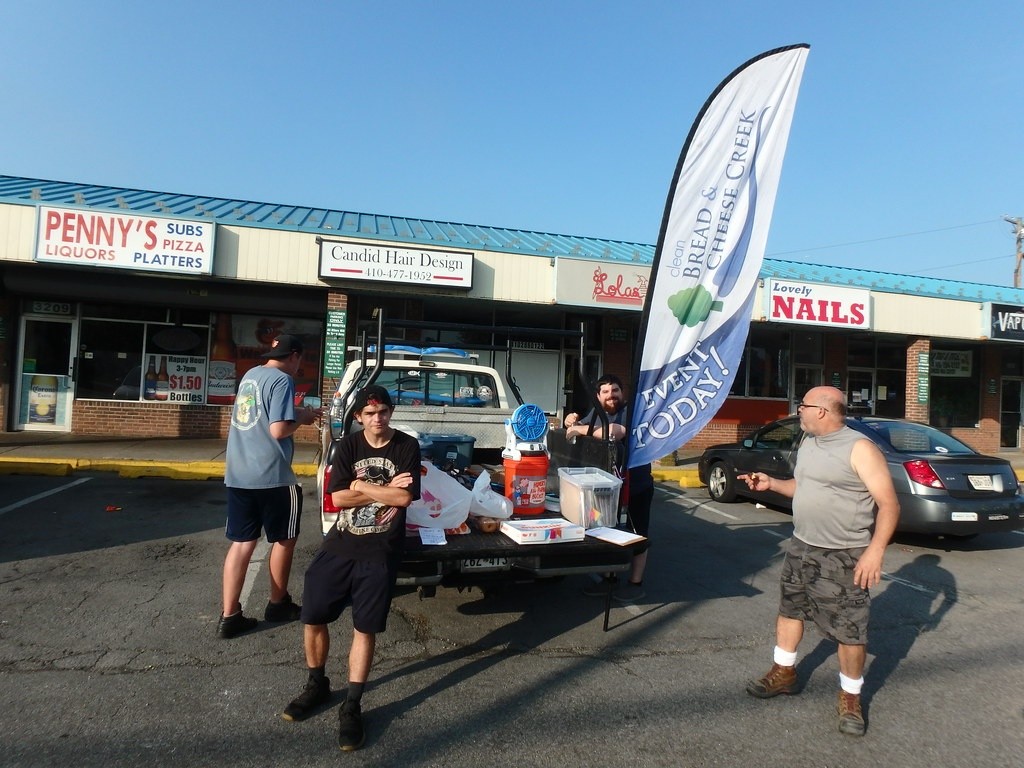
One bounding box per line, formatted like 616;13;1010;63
392;512;648;629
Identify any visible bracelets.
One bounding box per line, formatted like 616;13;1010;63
352;479;359;491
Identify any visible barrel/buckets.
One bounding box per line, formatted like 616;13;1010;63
502;451;549;515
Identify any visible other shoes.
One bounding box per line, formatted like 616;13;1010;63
614;580;646;601
583;578;618;597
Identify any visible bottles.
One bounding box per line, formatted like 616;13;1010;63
156;355;170;401
207;311;238;405
331;391;343;439
568;413;576;444
144;355;157;400
26;375;58;426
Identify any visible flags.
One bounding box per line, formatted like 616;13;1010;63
625;41;812;467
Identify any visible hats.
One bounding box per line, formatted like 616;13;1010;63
260;335;304;359
354;385;393;412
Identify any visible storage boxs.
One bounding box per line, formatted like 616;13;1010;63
500;518;585;546
556;465;624;529
388;424;475;472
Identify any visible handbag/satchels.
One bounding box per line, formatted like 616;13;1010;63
406;460;473;530
470;468;514;519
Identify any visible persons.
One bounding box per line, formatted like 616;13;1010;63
279;384;422;755
736;386;901;736
565;375;657;603
213;334;325;640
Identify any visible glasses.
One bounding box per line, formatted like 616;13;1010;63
797;404;829;413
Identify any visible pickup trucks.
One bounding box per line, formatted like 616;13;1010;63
315;359;632;598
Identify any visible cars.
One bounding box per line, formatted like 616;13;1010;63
112;363;141;400
698;415;1024;536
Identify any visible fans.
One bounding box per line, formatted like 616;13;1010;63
501;403;551;460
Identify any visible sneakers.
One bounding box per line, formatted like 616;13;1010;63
836;686;865;737
265;590;302;623
745;662;802;698
282;673;335;724
338;702;366;750
216;602;259;640
